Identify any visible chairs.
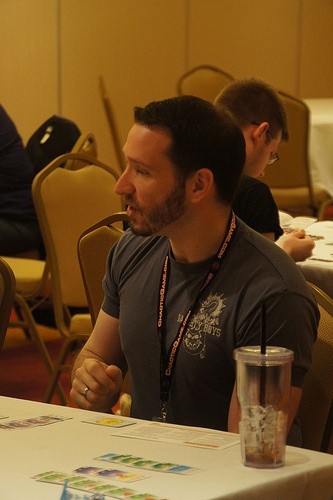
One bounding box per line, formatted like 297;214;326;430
177;65;333;221
0;76;138;417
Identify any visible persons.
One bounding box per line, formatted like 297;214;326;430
0;104;40;256
213;78;316;262
69;94;321;438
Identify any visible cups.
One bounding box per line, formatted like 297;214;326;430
233;345;293;469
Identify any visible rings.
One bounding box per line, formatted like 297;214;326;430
83;387;89;396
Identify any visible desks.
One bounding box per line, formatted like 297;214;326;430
303;97;333;204
275;219;333;295
0;396;333;500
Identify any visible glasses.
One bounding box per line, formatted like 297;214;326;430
252;121;279;164
